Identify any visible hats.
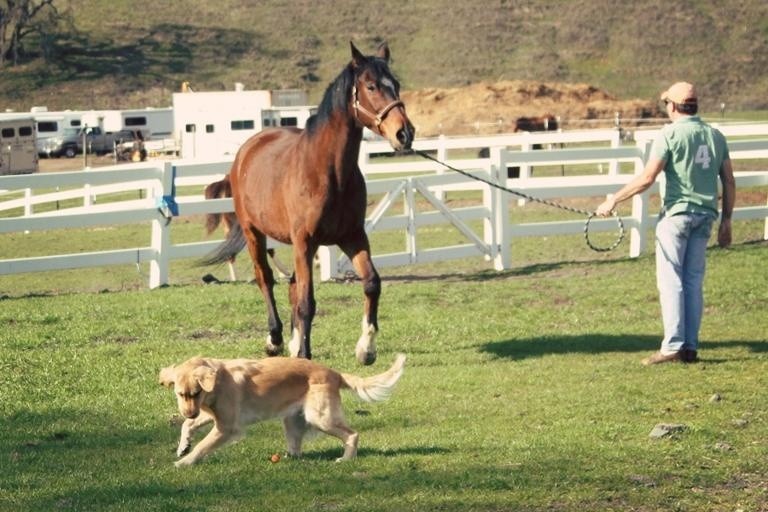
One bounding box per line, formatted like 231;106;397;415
661;82;696;105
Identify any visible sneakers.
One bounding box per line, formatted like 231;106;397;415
686;351;697;363
641;349;686;366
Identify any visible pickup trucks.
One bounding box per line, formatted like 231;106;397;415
37;125;132;159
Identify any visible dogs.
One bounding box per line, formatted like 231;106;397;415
158;353;407;468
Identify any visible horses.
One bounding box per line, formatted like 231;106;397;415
511;114;560;136
188;37;415;366
476;143;543;177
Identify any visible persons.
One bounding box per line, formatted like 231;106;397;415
593;79;738;368
